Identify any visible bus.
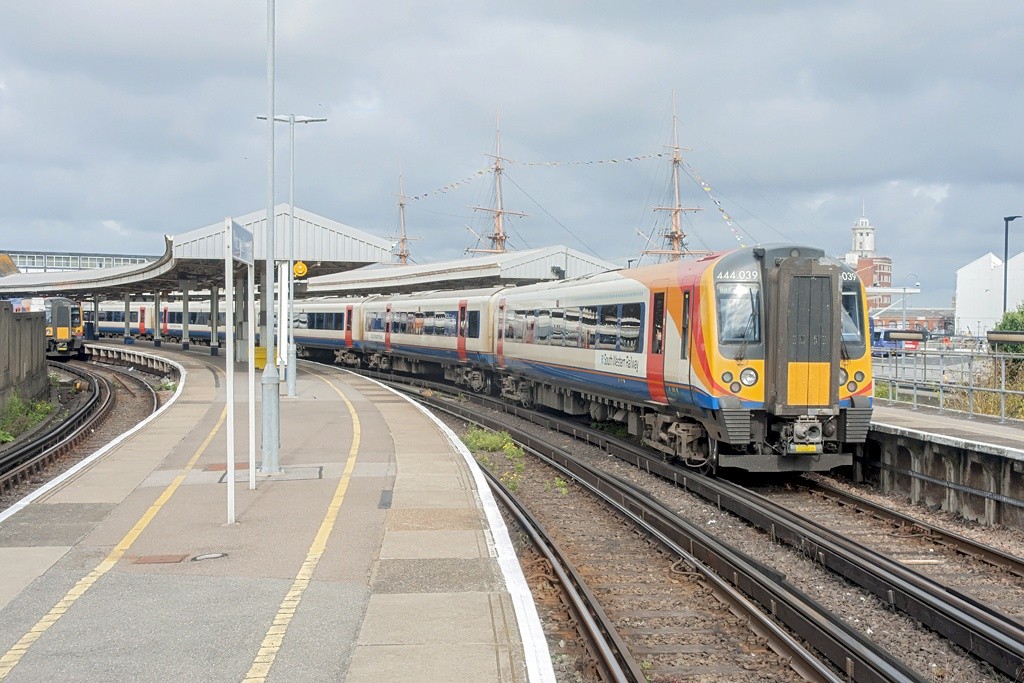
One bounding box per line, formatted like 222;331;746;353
869;327;924;357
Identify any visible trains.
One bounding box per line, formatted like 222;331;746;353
8;296;86;358
80;241;876;474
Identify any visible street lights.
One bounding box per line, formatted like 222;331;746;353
1002;215;1023;313
977;321;981;338
257;113;330;398
